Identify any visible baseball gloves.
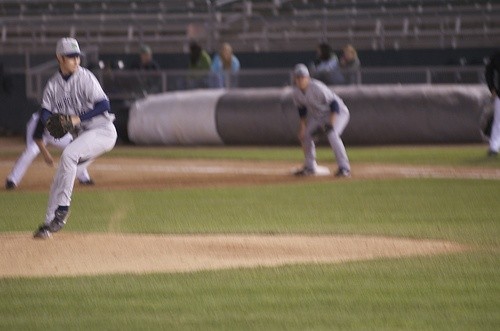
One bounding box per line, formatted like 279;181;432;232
48;113;72;138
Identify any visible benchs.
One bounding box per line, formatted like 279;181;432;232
0;0;500;54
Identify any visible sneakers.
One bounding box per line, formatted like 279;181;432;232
332;168;352;177
47;208;71;232
295;165;318;178
33;224;53;240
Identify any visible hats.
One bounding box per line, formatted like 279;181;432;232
56;37;83;59
293;61;311;81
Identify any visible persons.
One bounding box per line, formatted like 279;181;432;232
183;38;212;87
339;44;361;85
307;42;346;85
479;50;500;158
206;42;241;87
4;107;95;190
132;44;167;99
291;63;351;178
40;39;117;241
